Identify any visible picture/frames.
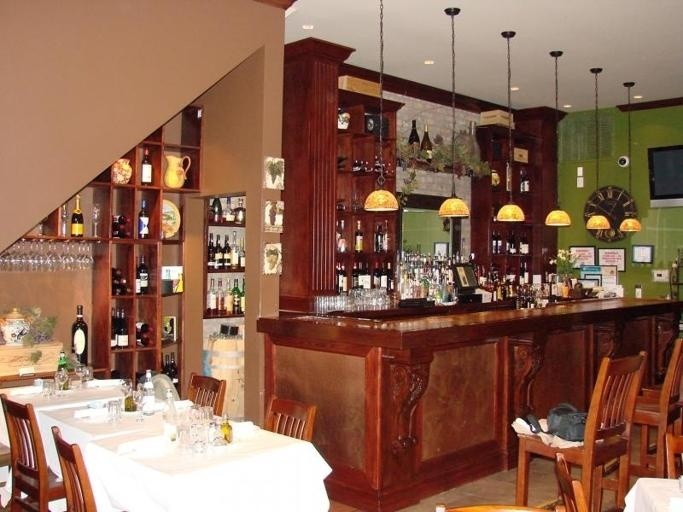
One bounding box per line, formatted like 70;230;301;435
569;244;654;286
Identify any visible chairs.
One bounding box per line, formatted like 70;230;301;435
266;395;317;442
641;384;670;465
186;373;226;416
598;338;683;478
0;393;65;512
52;426;97;512
516;351;647;508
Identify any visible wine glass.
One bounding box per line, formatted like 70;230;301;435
74;365;87;391
60;202;71;238
54;367;68;391
88;204;106;238
351;288;392;307
37;216;50;236
0;237;94;271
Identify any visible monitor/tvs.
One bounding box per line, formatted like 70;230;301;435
452;264;478;294
648;145;683;208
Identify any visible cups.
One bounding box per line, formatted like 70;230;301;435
43;379;54;397
107;399;120;419
311;295;346;311
174;404;213;446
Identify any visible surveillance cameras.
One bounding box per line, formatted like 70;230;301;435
618;156;629;168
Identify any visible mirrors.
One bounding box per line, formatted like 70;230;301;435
396;191;461;257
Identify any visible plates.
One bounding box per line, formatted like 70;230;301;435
162;197;181;239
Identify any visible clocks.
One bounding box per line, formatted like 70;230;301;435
583;185;638;242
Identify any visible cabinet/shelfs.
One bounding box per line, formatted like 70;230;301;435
83;106;204;195
335;88;405;294
204;195;245;318
471;124;534;276
17;214;107;241
91;189;185;401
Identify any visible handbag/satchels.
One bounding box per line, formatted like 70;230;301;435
547;406;588;441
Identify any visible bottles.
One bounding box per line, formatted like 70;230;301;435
208;412;232;442
336;153;394;296
70;304;90;365
160;351;179;386
111;266;131;296
454;120;482;165
548;274;557;303
491;168;529;287
135;321;155;347
490;282;512;302
142;147;151;185
111;212;131;239
406;119;434;164
134;253;148;294
57;350;70;390
512;283;536;310
138;200;150;240
205;194;247;317
70;194;84;236
165;388;176;423
111;305;129;350
396;249;493;299
142;369;155;416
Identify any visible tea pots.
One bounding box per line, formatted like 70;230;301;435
162;153;190;189
0;306;33;347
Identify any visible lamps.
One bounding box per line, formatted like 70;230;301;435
438;7;470;218
363;0;399;212
619;82;642;232
545;52;571;227
586;68;612;230
496;30;525;223
